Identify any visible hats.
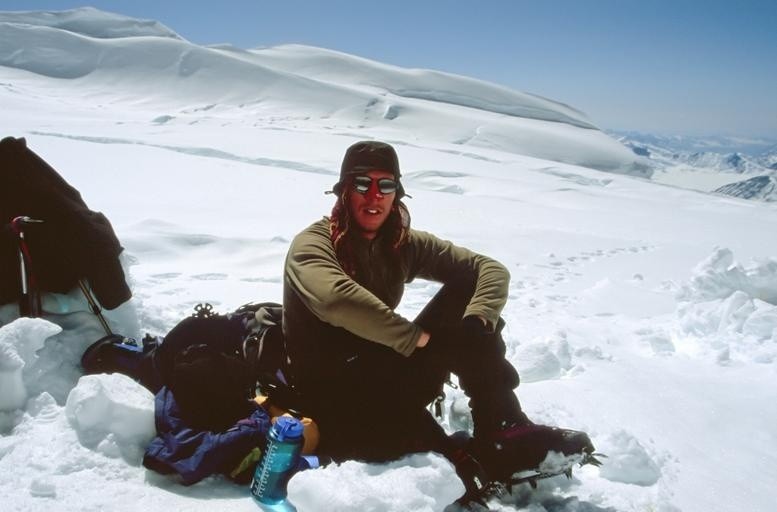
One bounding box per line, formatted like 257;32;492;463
333;141;405;198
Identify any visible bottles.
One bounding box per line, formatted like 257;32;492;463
249;417;306;507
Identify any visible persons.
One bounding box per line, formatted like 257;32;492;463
280;141;594;498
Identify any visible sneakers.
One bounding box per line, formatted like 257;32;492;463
446;401;596;478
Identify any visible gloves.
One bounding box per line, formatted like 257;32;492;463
424;316;488;359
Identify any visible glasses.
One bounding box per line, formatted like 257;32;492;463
351;176;399;194
